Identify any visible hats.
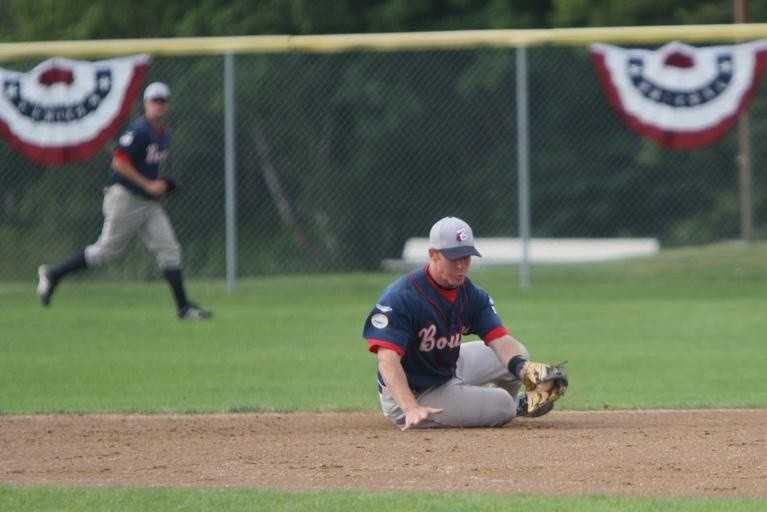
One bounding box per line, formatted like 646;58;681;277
430;217;481;260
144;82;170;101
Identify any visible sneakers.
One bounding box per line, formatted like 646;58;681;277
38;265;54;306
177;302;212;318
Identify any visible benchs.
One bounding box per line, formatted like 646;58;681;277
378;236;661;273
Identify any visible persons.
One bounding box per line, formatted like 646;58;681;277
33;81;214;319
361;214;567;432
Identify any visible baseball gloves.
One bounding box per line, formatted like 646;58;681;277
517;361;567;417
